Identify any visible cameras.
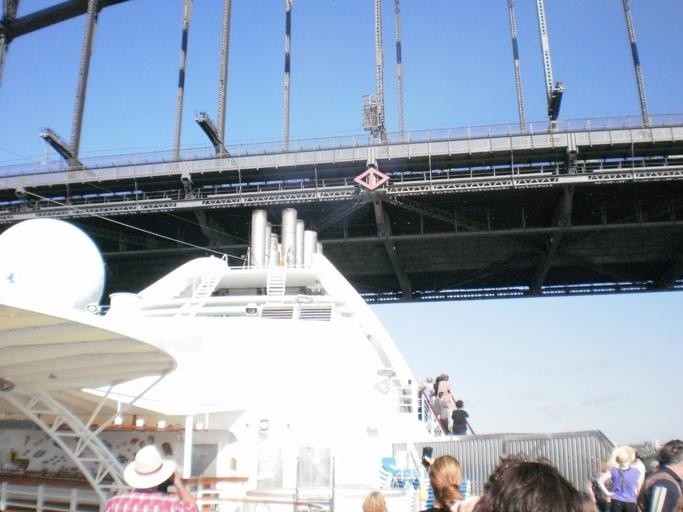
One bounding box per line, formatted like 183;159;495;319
158;472;176;491
423;446;434;466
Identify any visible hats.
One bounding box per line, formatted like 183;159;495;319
610;445;636;470
437;381;453;393
123;444;176;489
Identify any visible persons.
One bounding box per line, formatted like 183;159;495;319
403;374;469;435
471;453;585;512
578;491;599;512
363;491;389;512
586;439;683;512
103;445;199;512
419;455;464;512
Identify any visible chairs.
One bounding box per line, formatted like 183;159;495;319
292;445;336;512
